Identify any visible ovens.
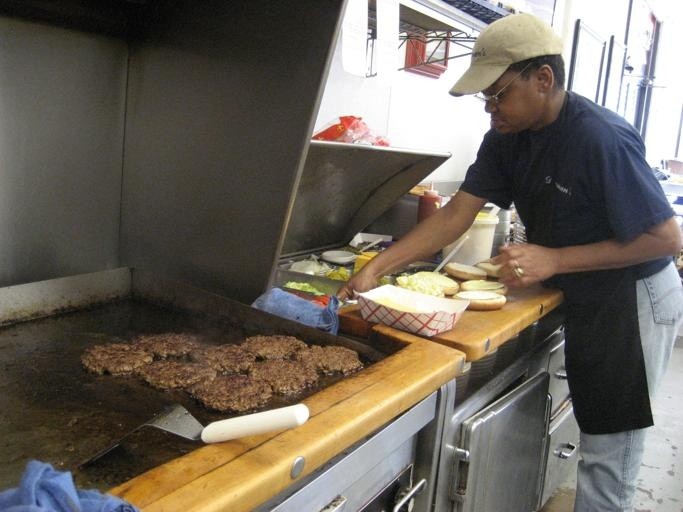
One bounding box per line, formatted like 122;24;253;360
269;388;451;512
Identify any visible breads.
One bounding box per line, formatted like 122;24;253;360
413;263;510;311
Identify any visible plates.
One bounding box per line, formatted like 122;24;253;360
322;250;354;263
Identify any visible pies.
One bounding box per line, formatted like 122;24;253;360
79;329;362;414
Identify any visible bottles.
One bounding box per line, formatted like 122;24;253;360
417;181;442;225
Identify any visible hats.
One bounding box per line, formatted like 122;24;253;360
448;12;564;98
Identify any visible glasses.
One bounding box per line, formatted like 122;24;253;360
472;61;535;105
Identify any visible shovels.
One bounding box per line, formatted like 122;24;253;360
79;403;309;471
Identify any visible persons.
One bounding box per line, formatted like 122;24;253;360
331;8;682;511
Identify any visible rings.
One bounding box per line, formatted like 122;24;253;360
509;259;520;270
510;268;523;280
